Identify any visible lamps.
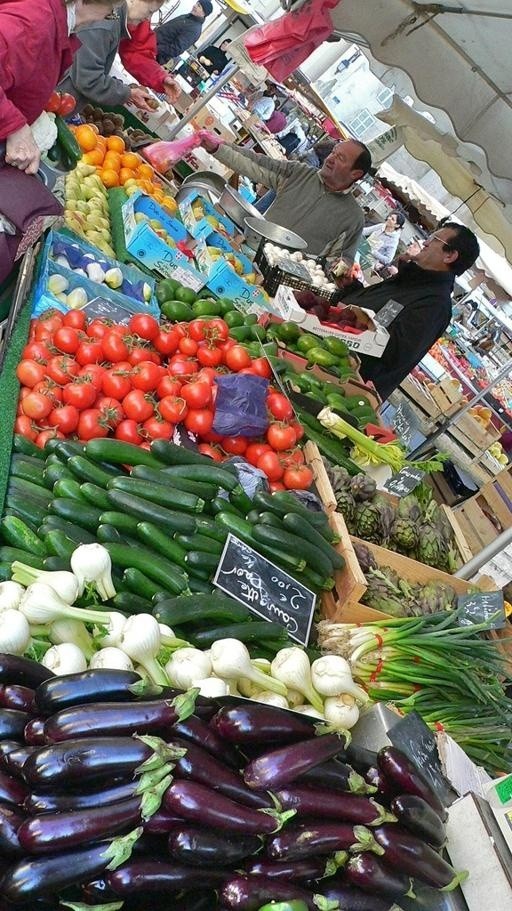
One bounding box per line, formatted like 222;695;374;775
333;47;362;77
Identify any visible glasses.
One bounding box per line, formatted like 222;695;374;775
429;231;452;249
106;1;120;21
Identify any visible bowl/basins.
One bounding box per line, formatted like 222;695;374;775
179;170;308;257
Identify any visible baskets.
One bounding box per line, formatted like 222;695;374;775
252;236;344;307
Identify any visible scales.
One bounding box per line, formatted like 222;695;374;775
444;771;512;911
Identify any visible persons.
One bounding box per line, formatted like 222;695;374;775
0;0;495;405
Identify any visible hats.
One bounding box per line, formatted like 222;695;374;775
198;0;212;16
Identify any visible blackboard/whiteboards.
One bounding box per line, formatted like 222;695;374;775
387;708;459;810
211;532;318;648
79;296;136;324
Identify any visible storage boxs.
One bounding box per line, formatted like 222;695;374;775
111;70;237;172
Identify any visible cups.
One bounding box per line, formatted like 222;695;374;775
332;258;350;278
